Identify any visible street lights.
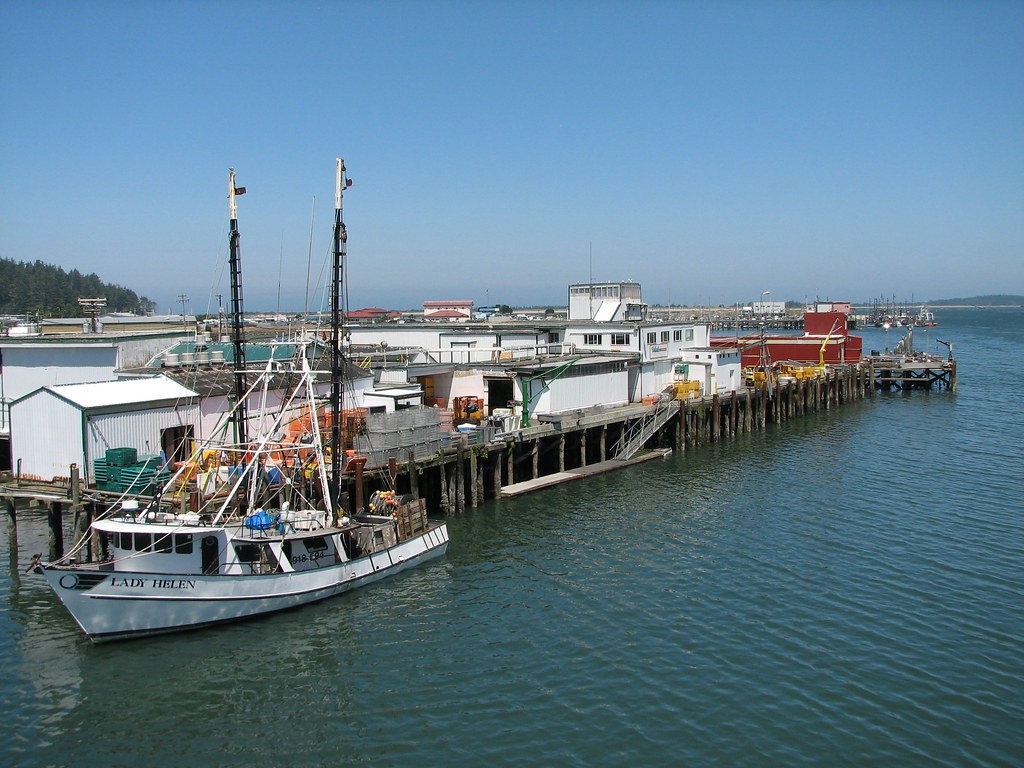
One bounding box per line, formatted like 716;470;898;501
758;291;770;370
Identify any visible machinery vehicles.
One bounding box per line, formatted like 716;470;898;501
744;318;840;388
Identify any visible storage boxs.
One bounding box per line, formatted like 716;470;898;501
171;400;521;490
93;447;171;495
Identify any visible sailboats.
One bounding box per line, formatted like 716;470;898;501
32;157;449;646
